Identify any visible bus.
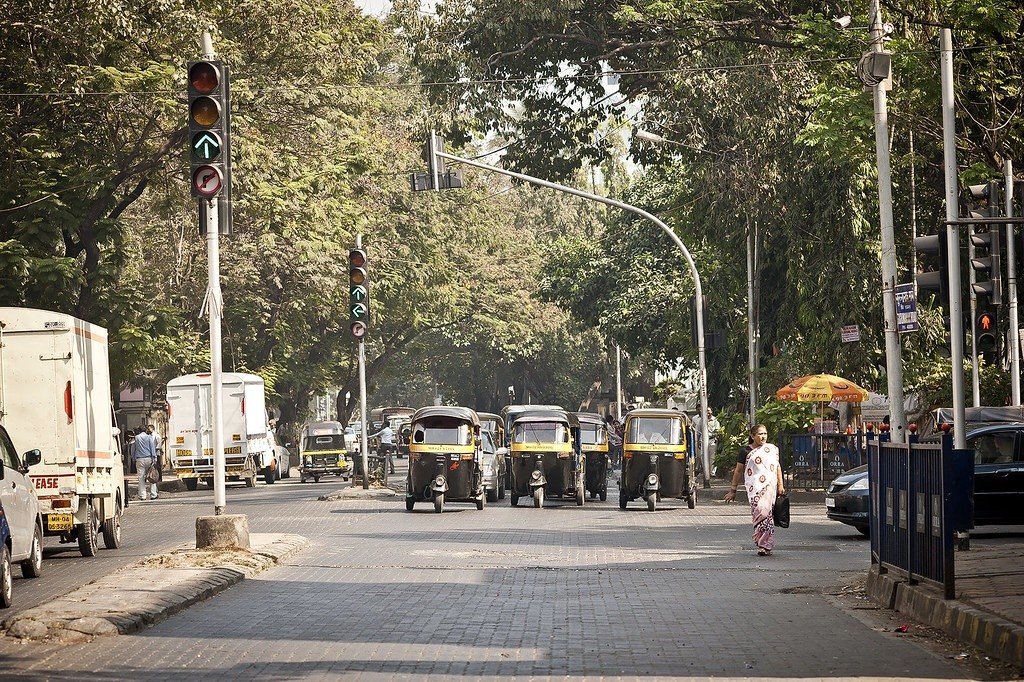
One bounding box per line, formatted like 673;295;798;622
371;407;418;452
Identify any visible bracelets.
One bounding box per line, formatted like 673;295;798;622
730;489;735;493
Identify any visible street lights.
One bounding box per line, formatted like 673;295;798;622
636;129;760;428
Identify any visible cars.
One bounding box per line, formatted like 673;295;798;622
395;422;411;458
824;420;1024;535
0;424;44;578
344;427;360;461
274;433;291;480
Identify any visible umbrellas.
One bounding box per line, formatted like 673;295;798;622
777;372;870;483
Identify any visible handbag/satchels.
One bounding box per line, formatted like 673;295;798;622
146;463;159;484
774;492;790;528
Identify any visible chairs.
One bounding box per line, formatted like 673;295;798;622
975;438;999;463
995;436;1014;463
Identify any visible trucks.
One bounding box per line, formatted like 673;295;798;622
0;307;137;556
166;371;278;491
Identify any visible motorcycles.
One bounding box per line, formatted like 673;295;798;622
299;401;697;512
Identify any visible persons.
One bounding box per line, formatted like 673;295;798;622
723;424;785;554
604;405;721;477
123;425;163;501
367;421;395;475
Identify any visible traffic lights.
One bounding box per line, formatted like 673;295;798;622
349;248;368;320
186;61;226;164
977;311;998;352
968;180;1002;305
915;221;949;315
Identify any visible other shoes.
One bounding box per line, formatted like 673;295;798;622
151;495;159;500
757;547;773;556
388;472;395;474
